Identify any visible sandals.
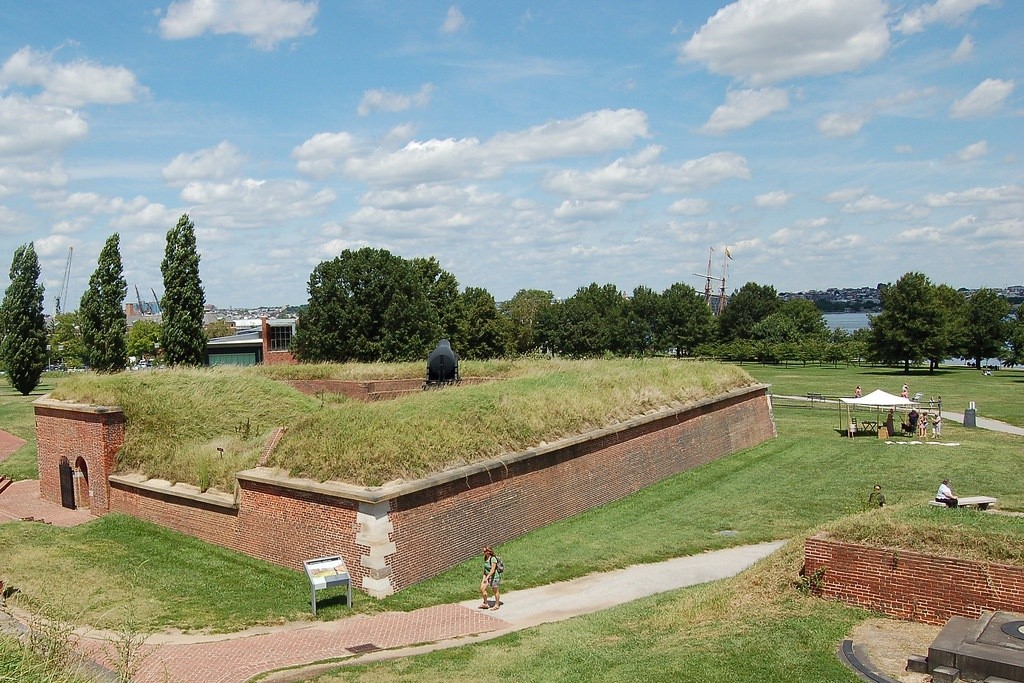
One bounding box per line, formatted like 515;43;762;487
478;604;488;609
491;605;500;611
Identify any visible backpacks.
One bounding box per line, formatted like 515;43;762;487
490;555;504;573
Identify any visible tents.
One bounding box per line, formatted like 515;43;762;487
838;388;921;439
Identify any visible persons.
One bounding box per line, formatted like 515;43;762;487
478;544;502;611
854;385;862;398
900;382;909;398
935;478;958;508
867;484;887;509
887;408;896;437
908;396;942;440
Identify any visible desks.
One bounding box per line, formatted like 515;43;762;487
862;421;878;434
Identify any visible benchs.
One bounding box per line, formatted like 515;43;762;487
927;615;978;674
929;495;997;511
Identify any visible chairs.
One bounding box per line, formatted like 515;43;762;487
900;423;916;436
851;418;863;434
967;362;972;367
